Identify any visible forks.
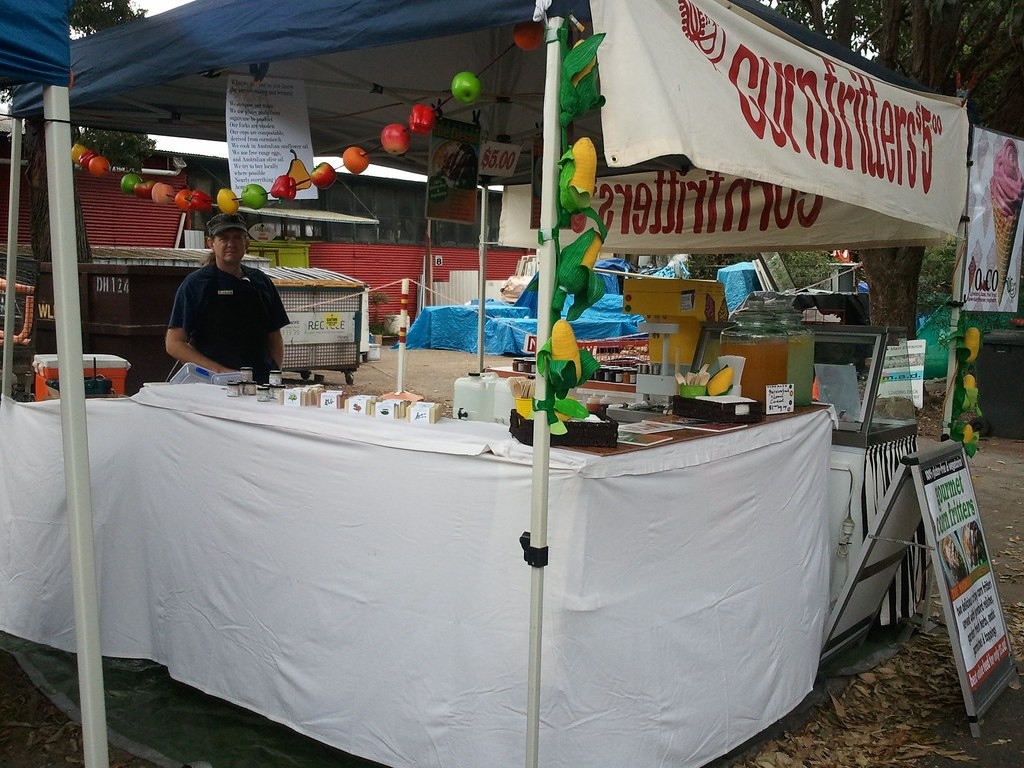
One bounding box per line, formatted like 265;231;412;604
675;364;709;386
508;379;535;399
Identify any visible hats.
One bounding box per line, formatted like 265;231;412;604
207;212;247;237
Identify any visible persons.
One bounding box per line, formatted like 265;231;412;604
165;214;291;386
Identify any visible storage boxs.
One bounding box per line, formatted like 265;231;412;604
170;364;240;384
671;394;763;424
509;408;619;447
32;353;131;402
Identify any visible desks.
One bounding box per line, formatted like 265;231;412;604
142;383;855;768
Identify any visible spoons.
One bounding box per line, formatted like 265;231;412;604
700;373;709;386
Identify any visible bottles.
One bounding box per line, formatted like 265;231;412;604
599;395;612;414
452;372;497;422
719;292;815;407
586;394;600;414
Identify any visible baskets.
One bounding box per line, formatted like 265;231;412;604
672;395;762;423
510;409;618;449
576;337;649;364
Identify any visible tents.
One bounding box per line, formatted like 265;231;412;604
0;0;110;768
0;2;970;768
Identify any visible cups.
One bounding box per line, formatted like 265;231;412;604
515;398;532;420
678;383;707;399
